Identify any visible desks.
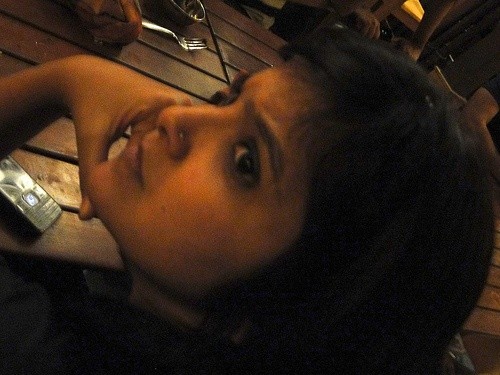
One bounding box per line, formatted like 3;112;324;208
1;0;498;340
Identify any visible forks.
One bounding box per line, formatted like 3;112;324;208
141;16;209;50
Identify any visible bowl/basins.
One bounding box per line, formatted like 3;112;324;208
164;0;206;25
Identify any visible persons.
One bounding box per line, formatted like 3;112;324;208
0;24;500;375
267;0;461;64
461;74;500;185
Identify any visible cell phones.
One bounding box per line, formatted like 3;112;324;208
1;155;63;235
